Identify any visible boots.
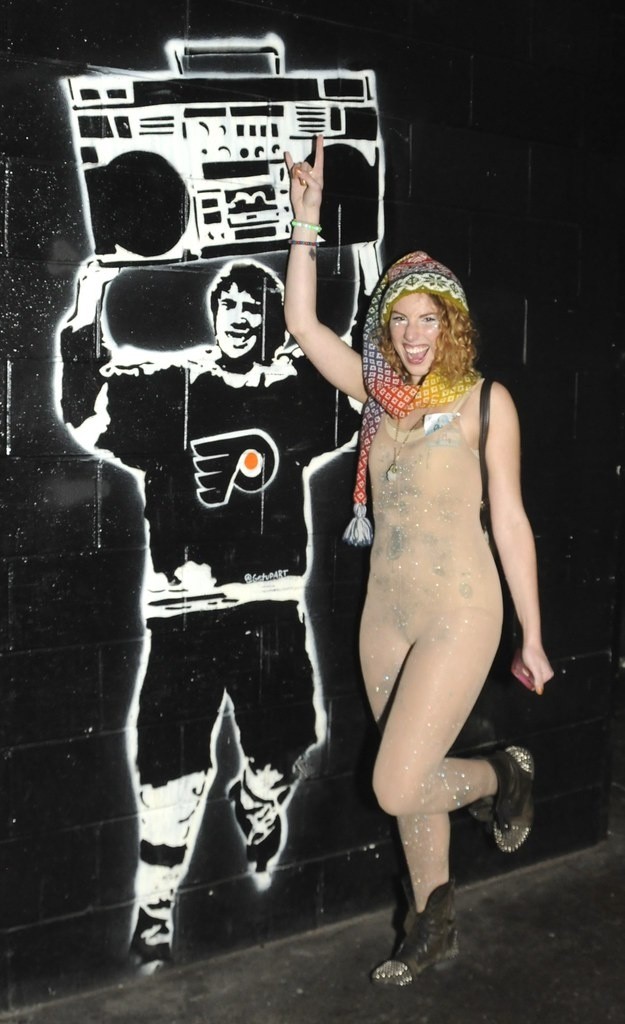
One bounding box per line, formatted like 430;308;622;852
468;744;535;854
370;869;460;992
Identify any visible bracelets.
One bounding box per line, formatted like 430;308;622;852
288;239;318;245
291;219;323;233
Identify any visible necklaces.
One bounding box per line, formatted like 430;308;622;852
387;405;433;483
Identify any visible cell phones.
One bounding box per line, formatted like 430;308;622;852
510;647;535;691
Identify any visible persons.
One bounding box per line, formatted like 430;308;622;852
284;132;559;985
